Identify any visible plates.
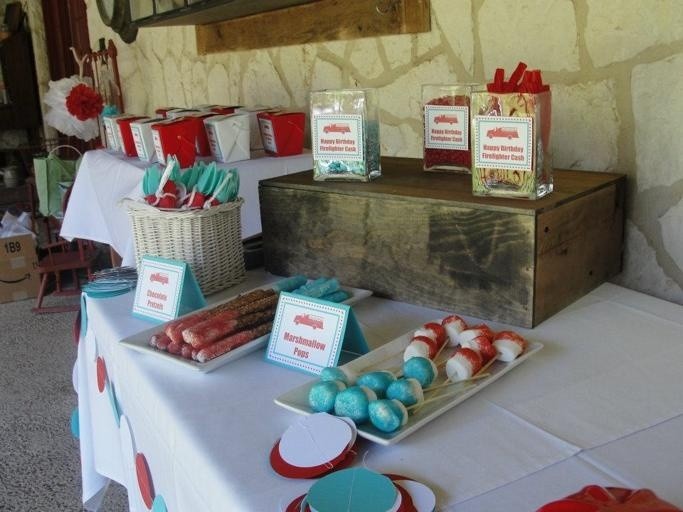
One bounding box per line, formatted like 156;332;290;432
120;277;373;373
273;318;544;446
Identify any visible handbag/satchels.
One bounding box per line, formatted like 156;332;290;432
33;155;79;218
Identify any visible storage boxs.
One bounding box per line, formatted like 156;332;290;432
255;164;625;329
0;234;40;304
103;103;307;169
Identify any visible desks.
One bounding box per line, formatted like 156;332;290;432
58;148;314;256
72;269;681;512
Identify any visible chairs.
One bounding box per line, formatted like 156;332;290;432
33;186;96;313
